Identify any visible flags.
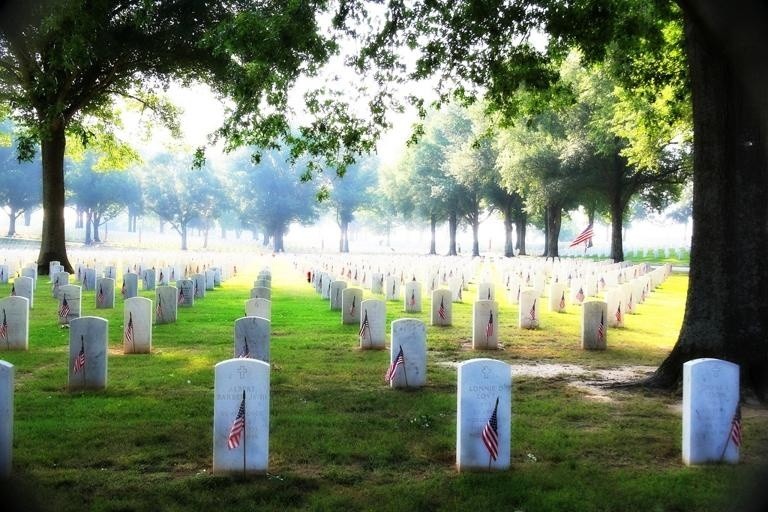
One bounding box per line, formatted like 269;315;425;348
236;345;250;359
383;351;403;382
0;260;218;376
226;399;245;450
568;224;592;248
307;261;651;339
731;405;744;448
481;409;499;462
